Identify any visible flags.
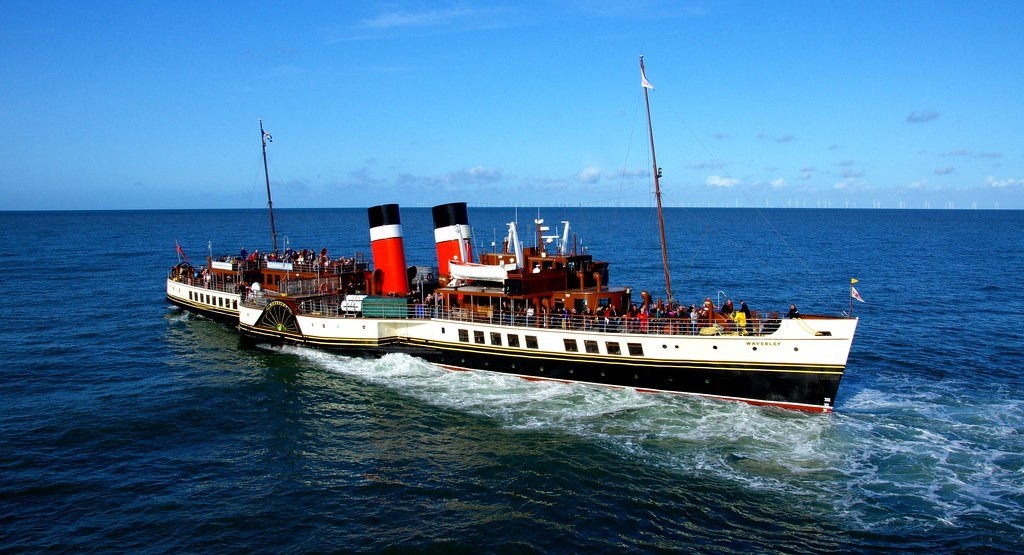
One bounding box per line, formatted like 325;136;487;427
851;285;866;303
261;127;274;142
177;243;189;261
641;65;655;91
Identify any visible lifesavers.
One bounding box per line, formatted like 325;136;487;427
320;283;327;294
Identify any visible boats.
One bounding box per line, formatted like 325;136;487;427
165;53;865;416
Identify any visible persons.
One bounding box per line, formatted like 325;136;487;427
788;304;802;319
170;245;458;319
491;298;782;336
